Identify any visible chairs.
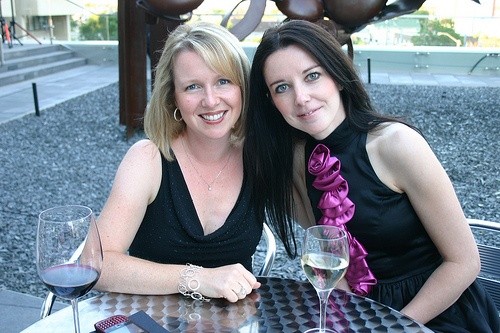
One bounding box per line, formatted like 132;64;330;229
40;220;277;322
467;219;500;311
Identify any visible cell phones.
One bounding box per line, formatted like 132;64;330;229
95;315;150;333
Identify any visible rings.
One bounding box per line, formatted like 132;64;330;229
236;287;246;295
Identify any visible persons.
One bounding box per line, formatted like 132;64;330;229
79;21;263;303
247;19;500;333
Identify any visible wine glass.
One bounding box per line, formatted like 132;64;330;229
36;205;103;333
301;225;350;333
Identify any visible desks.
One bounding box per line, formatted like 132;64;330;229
20;276;435;333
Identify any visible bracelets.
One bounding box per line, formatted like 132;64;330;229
178;262;212;301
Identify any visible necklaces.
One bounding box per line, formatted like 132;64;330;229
180;132;232;190
